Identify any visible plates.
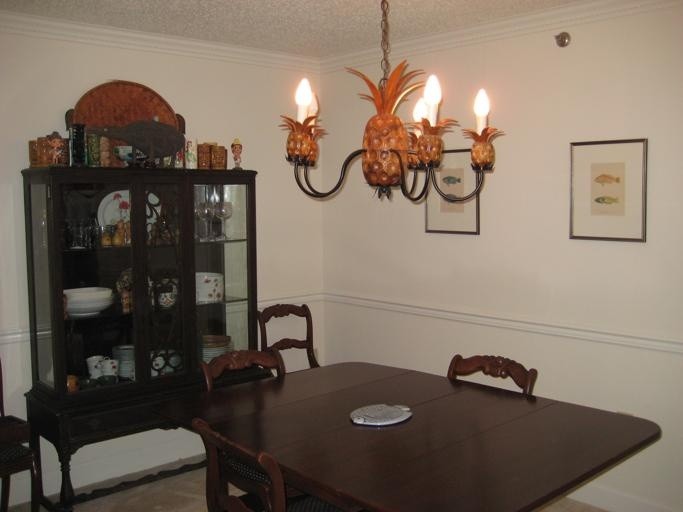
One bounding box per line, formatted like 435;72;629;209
111;333;232;382
63;285;115;319
194;271;225;305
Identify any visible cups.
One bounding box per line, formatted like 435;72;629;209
28;133;71;169
196;141;231;170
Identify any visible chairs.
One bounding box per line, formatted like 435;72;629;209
192;419;334;511
448;354;537;396
197;348;286;390
256;303;320;376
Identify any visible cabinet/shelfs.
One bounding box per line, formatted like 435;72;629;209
20;163;276;511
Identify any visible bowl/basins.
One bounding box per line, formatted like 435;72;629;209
76;354;121;392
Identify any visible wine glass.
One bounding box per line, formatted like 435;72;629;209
214;200;235;243
196;200;216;243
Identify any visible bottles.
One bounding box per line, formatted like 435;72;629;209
173;133;200;169
67;120;114;169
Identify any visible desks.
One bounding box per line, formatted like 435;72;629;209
160;361;576;510
149;362;660;511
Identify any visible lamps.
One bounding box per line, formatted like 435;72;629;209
278;0;507;204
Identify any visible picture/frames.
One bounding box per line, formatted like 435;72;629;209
424;148;482;236
568;137;649;243
